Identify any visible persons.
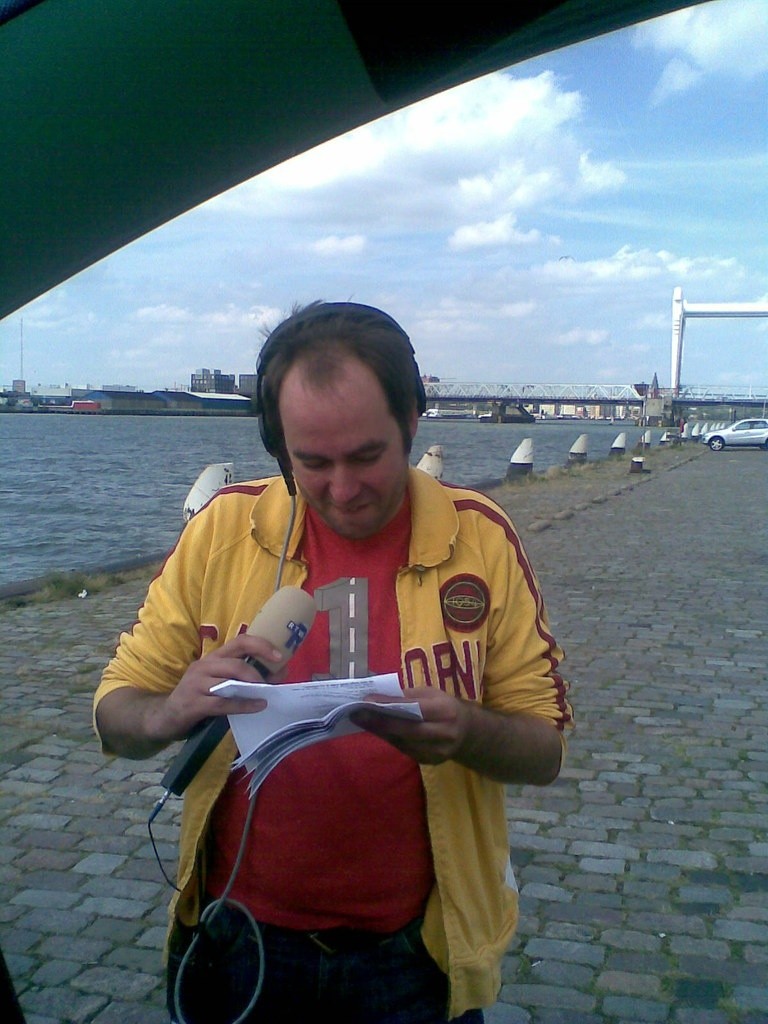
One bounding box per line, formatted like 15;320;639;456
92;301;578;1024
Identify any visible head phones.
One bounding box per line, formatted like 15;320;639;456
255;303;427;476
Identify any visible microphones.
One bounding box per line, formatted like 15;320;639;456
161;584;317;796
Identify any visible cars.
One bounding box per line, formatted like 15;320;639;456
702;418;768;451
